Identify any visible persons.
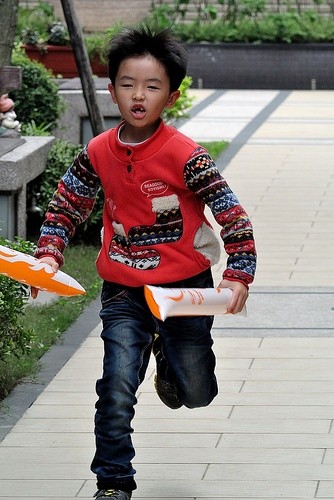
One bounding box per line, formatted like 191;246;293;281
30;22;257;499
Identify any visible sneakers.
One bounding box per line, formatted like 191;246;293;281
151;334;183;409
92;488;131;500
